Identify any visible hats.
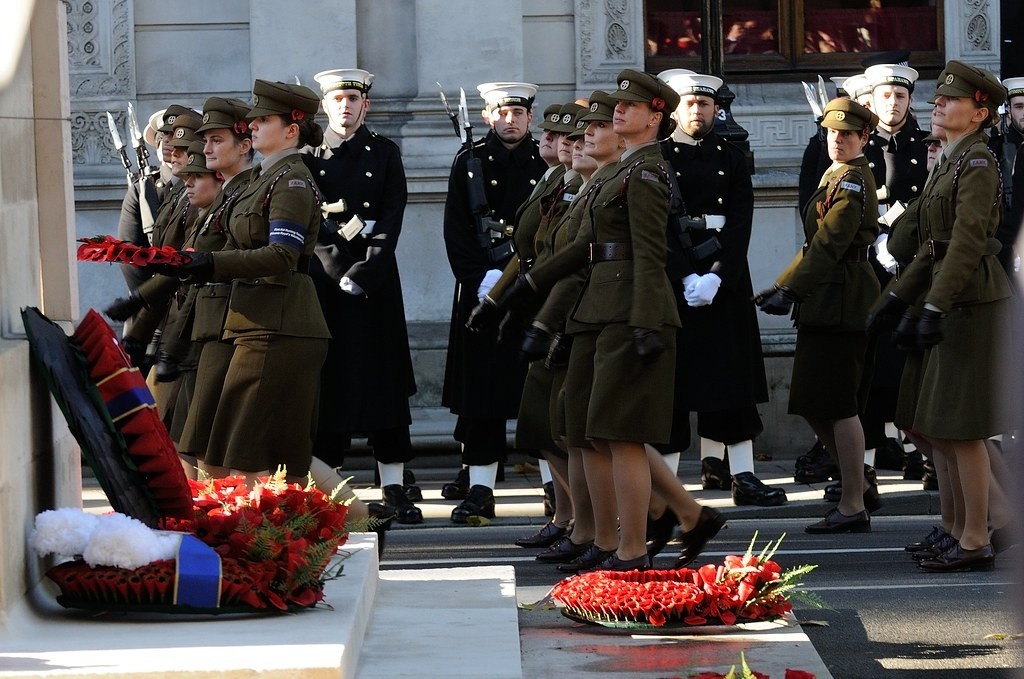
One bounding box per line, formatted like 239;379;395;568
820;60;1024;141
192;96;253;137
476;83;539;113
244;78;320;122
538;67;724;139
315;68;375;98
139;105;215;173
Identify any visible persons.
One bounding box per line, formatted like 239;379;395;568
750;61;1024;574
442;67;788;575
101;68;424;562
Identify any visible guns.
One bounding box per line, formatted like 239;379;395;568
106;101;163;245
434;80;506;251
802;77;835;150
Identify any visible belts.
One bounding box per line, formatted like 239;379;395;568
686;214;725;229
290;255;309;275
927;238;1002;255
588;242;633;263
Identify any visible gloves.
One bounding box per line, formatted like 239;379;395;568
119;338;146;378
679;271;721;307
464;277;661;372
337;277;363;296
155;350;178;386
476;268;501;302
101;296;140;322
165;248;216;285
751;287;794;315
865;233;947;347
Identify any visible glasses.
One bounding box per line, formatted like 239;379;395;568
924;142;942;148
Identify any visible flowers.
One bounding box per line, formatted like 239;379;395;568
545;528;838;632
20;305;391;621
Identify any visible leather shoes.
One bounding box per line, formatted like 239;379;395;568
442;436;1024;570
363;465;425;558
